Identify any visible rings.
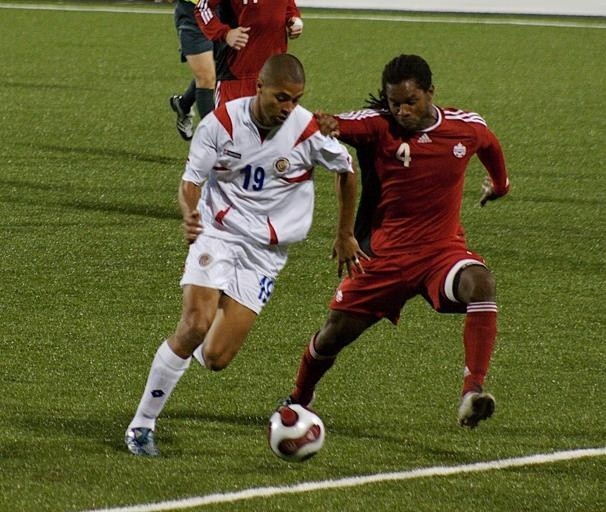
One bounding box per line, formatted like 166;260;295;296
352;256;359;266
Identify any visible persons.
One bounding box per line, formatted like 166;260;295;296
171;0;216;140
194;0;303;110
280;54;509;428
125;54;371;457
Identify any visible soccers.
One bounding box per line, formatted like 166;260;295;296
267;406;326;461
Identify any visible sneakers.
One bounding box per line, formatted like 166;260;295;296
455;389;498;431
125;426;161;458
170;92;195;140
